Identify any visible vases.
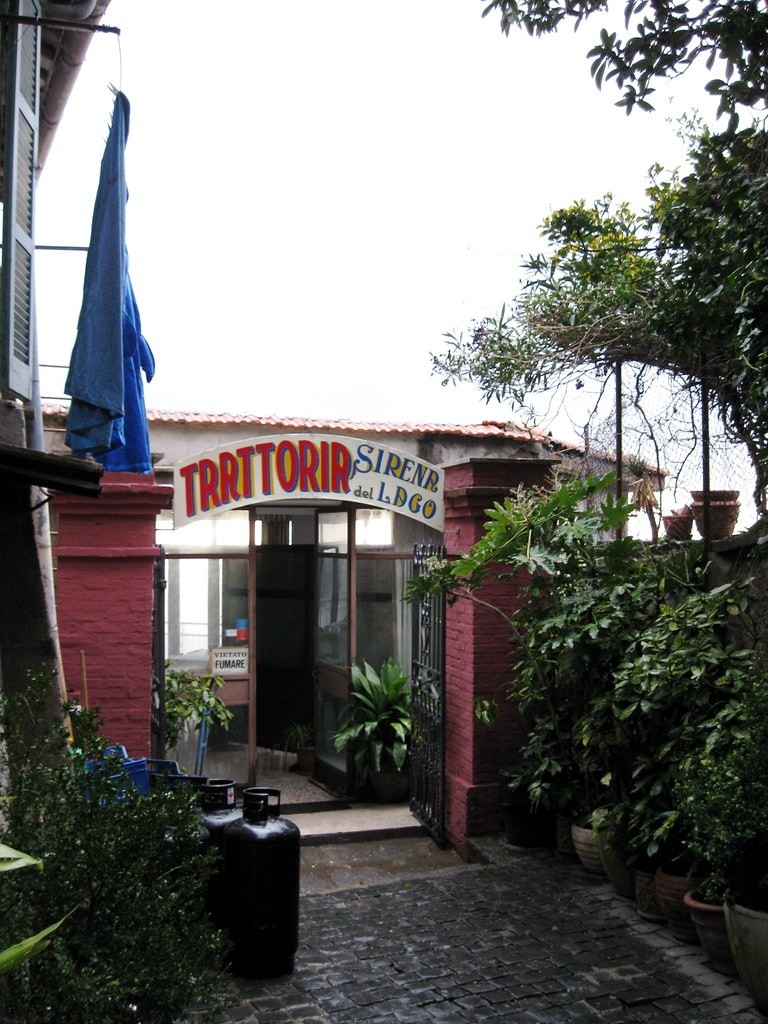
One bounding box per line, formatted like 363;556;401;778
690;501;741;541
691;491;740;503
662;516;694;541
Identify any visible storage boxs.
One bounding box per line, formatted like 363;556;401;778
75;745;207;810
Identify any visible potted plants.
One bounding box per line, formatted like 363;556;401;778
273;722;315;772
329;658;416;803
401;471;768;1018
